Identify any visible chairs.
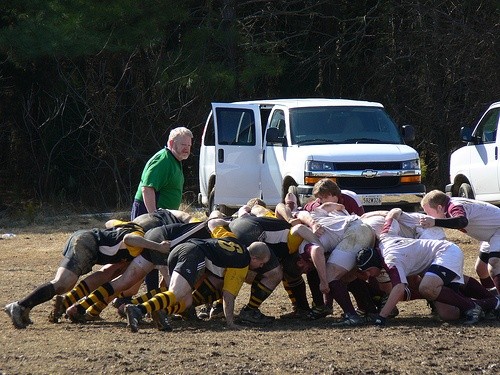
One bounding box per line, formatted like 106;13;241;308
341;114;362;132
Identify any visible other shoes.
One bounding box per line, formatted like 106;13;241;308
48;295;63;322
302;305;330;320
124;304;143;332
153;311;171;331
331;307;400;326
239;308;275;323
66;305;86;323
280;312;300;320
200;305;224;319
2;301;31;329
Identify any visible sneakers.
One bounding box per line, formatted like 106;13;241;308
463;301;481;324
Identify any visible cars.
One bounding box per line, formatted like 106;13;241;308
445;102;500;206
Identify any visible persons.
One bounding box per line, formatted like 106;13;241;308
4;126;500;334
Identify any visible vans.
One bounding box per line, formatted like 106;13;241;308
198;99;427;212
